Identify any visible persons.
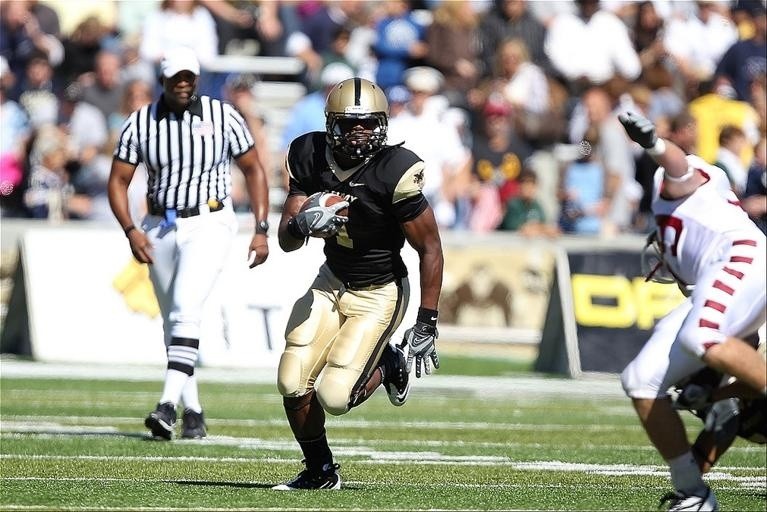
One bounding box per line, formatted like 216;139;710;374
674;330;767;474
270;77;444;491
107;49;268;438
618;110;767;512
0;0;767;235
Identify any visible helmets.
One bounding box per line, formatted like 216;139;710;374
324;79;389;168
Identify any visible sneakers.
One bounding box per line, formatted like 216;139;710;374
145;402;208;439
383;338;410;406
662;487;717;512
271;470;341;490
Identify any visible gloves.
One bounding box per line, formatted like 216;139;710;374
617;111;657;148
401;321;439;377
287;192;349;238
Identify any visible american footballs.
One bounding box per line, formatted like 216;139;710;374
298;192;349;238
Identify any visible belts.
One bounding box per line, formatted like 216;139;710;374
150;203;225;218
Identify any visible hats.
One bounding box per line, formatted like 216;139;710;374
154;35;201;79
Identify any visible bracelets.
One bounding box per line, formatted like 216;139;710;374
417;307;438;326
288;218;305;240
